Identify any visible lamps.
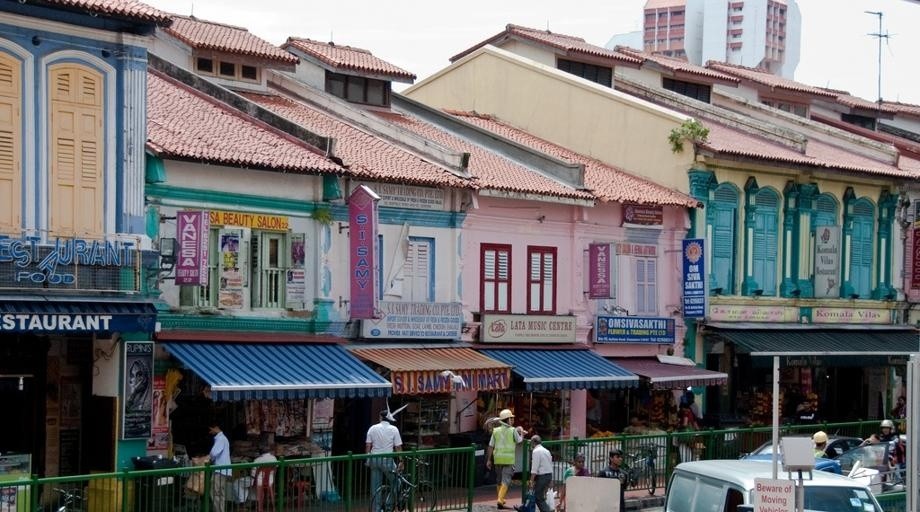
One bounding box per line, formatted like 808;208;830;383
160;238;182;264
0;374;33;391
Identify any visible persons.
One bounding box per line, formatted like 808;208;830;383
204;421;279;510
892;395;906;418
127;356;151;414
556;455;588;512
514;435;555;512
813;419;907;494
586;393;645;438
792;399;822;433
678;401;699;462
486;409;524;509
533;402;553;442
597;449;630;511
365;410;405;511
686;392;703;460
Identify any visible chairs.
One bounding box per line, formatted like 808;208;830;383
249;466;277;511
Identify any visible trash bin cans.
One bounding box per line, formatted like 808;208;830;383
450;430;492;488
713;413;745;460
132;455;182;512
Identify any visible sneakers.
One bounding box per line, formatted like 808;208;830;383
514;505;521;512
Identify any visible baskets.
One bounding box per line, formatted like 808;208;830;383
642;447;657;457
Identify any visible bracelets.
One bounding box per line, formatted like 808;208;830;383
399;459;403;463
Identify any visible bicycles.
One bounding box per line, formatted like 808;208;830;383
52;487;89;512
616;442;658;494
369;456;438;512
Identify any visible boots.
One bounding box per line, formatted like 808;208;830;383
496;484;508;508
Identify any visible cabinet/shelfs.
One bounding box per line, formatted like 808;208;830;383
400;393;451;438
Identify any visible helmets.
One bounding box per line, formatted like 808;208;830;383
499;409;515;420
813;431;827;443
880;420;895;433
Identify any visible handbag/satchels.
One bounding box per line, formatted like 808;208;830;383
522;494;535;512
187;468;205;495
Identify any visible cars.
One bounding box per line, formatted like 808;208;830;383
662;432;906;512
